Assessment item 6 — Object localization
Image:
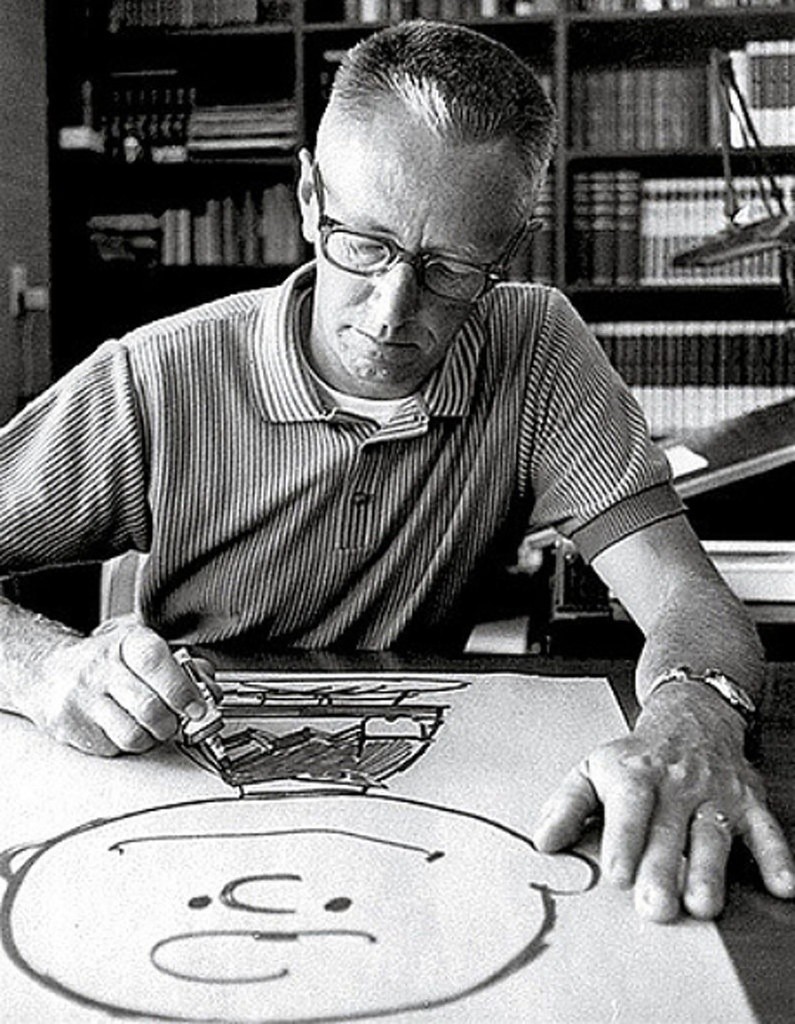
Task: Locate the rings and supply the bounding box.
[693,807,732,843]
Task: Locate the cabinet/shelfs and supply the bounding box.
[44,0,795,530]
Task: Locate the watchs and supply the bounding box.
[648,667,756,733]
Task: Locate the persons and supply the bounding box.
[0,27,795,923]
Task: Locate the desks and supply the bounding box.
[0,651,795,1024]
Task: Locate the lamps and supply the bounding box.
[674,46,795,268]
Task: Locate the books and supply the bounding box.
[53,1,795,440]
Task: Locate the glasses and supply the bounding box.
[310,165,530,305]
